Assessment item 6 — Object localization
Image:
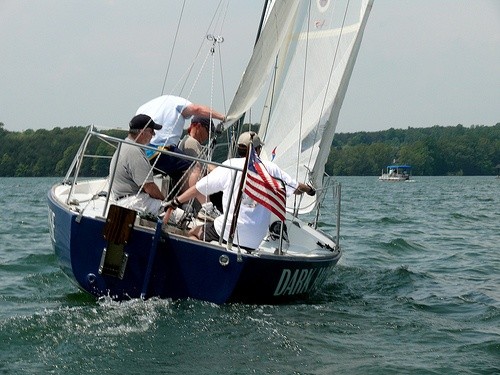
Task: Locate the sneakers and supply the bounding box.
[197,206,222,222]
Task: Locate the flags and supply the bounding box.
[243,142,287,222]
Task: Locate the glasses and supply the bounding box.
[149,130,155,136]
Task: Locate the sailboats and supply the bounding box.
[46,0,374,304]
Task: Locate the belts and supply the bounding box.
[117,190,143,201]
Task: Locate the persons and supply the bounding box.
[109,114,207,228]
[135,95,227,219]
[163,131,316,253]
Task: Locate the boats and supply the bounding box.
[378,165,412,181]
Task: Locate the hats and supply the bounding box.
[191,114,215,134]
[237,131,264,150]
[129,113,163,130]
